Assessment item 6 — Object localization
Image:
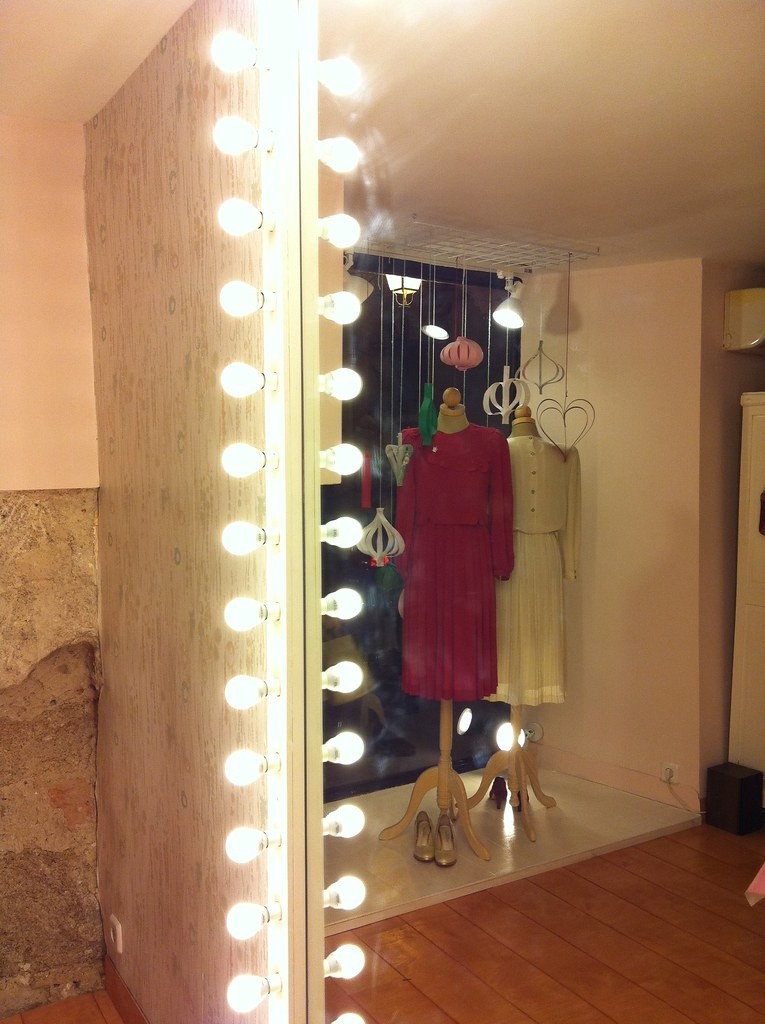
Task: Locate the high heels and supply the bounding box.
[517,791,529,812]
[414,811,435,862]
[435,815,457,865]
[489,777,507,808]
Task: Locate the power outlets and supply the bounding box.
[658,762,678,785]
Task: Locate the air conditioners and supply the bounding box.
[723,288,765,356]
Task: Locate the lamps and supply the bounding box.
[496,722,543,752]
[420,287,458,339]
[384,274,420,305]
[492,273,526,328]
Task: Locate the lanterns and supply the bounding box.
[439,335,484,372]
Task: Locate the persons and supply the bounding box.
[483,404,583,708]
[392,387,515,706]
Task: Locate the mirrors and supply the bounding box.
[304,0,765,1024]
